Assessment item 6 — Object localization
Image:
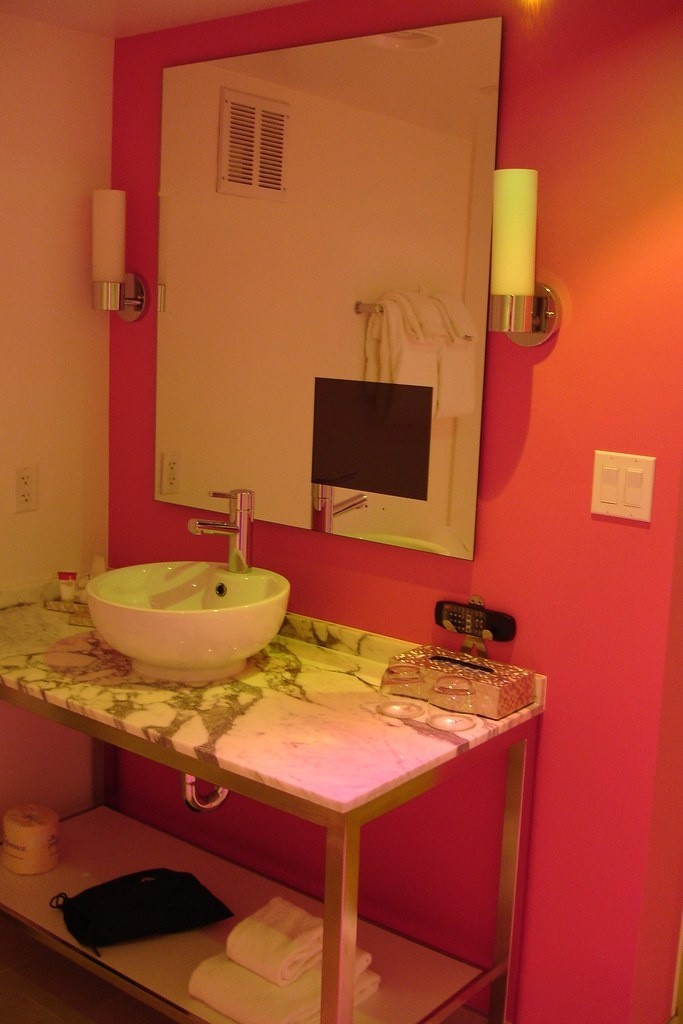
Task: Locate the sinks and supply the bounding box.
[88,561,290,682]
[345,535,450,560]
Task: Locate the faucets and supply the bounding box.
[189,488,254,573]
[310,484,368,533]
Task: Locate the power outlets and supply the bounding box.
[14,465,38,512]
[160,451,180,494]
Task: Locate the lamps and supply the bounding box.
[488,167,561,347]
[92,190,147,322]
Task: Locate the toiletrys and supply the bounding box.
[57,570,78,603]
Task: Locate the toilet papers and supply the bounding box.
[3,802,60,876]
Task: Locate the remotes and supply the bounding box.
[434,600,515,642]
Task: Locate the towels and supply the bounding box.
[365,290,481,416]
[188,896,382,1022]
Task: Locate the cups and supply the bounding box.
[378,665,427,718]
[427,675,477,731]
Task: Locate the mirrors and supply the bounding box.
[156,16,502,561]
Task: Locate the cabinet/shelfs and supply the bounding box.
[0,603,546,1024]
